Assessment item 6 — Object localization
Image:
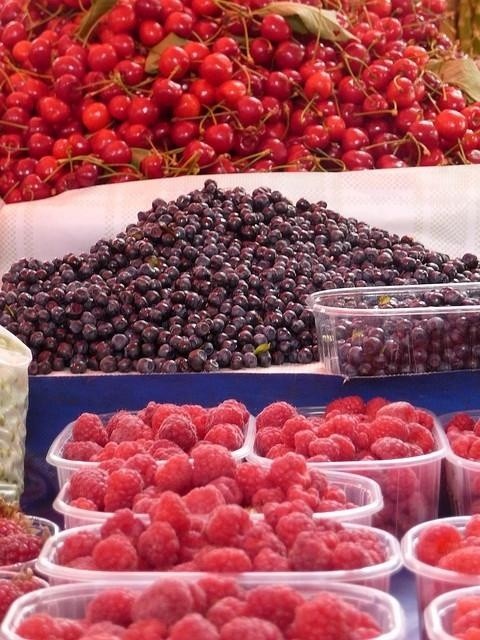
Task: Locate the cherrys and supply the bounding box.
[0,0,480,204]
